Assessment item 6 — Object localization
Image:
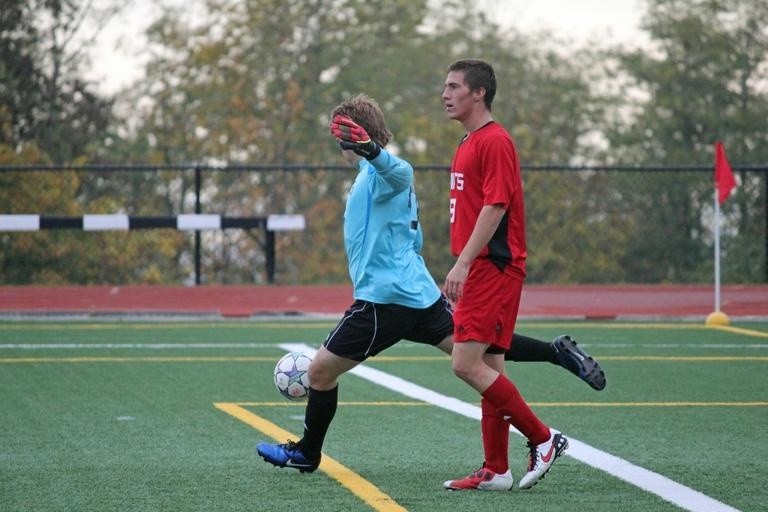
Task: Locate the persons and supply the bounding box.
[441,59,569,492]
[254,95,609,475]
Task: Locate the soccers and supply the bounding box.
[274,352,315,400]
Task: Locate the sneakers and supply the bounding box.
[443,466,515,491]
[255,439,325,473]
[546,332,607,392]
[518,423,570,490]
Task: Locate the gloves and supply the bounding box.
[329,112,381,162]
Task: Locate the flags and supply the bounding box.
[715,141,739,206]
